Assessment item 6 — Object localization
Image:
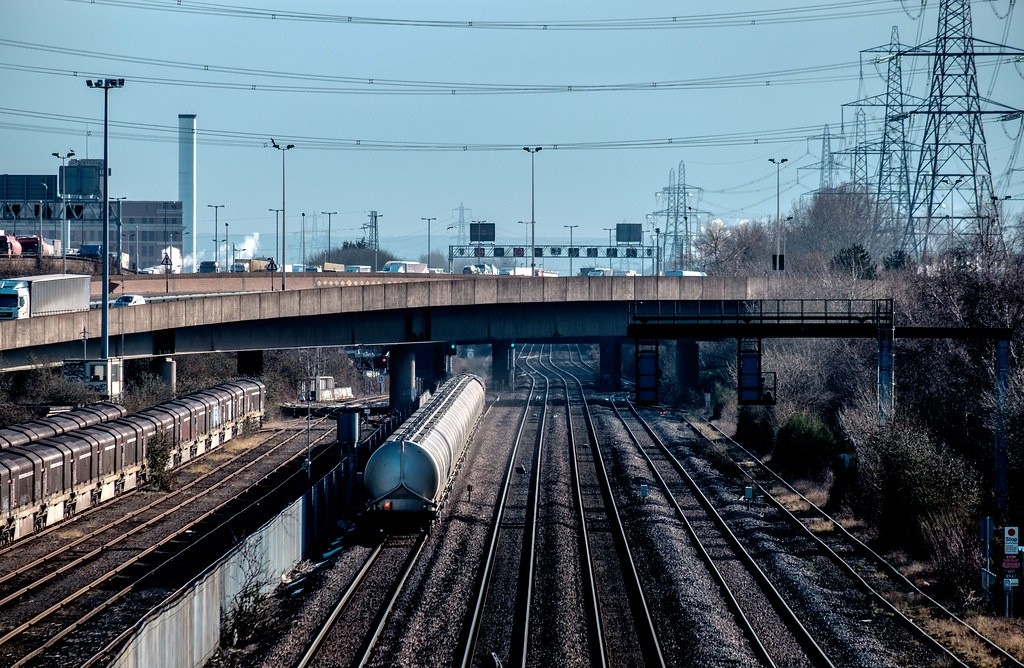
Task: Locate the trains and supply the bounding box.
[355,369,488,535]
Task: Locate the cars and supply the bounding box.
[112,294,146,309]
[230,263,246,272]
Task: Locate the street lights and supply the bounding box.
[160,201,190,274]
[684,216,687,271]
[521,145,544,277]
[655,227,660,274]
[235,248,246,263]
[367,214,383,271]
[603,228,617,268]
[207,204,226,273]
[52,152,76,275]
[768,157,793,300]
[471,220,487,265]
[86,78,125,360]
[688,206,692,270]
[321,211,338,263]
[421,217,436,268]
[564,225,579,277]
[268,144,295,272]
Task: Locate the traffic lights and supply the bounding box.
[379,356,387,365]
[447,342,457,356]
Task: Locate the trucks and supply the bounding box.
[0,235,132,321]
[292,260,643,277]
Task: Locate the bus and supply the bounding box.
[197,260,220,274]
